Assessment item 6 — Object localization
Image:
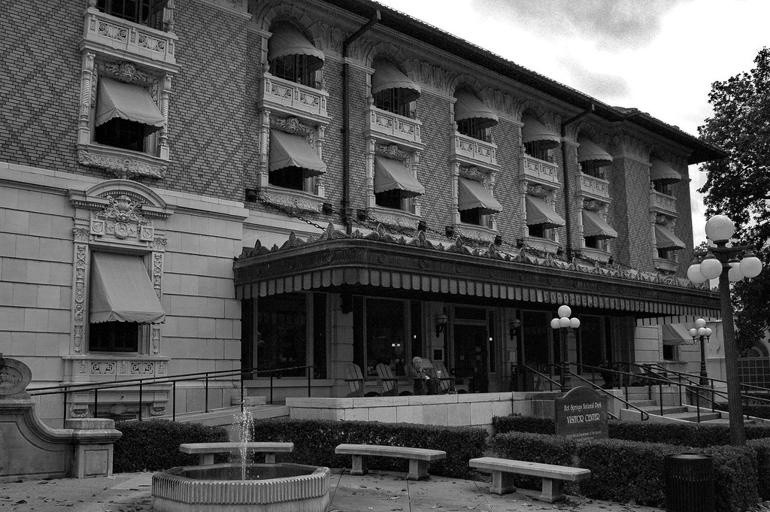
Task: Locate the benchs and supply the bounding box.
[179,442,294,465]
[469,457,591,503]
[335,443,446,479]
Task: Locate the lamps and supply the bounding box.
[436,315,449,337]
[510,319,521,340]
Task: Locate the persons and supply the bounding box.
[410,356,434,394]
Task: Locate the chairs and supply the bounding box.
[344,361,458,397]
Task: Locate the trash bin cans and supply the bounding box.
[662,451,714,512]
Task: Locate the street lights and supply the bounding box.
[686,214,763,445]
[689,317,713,385]
[551,305,581,393]
